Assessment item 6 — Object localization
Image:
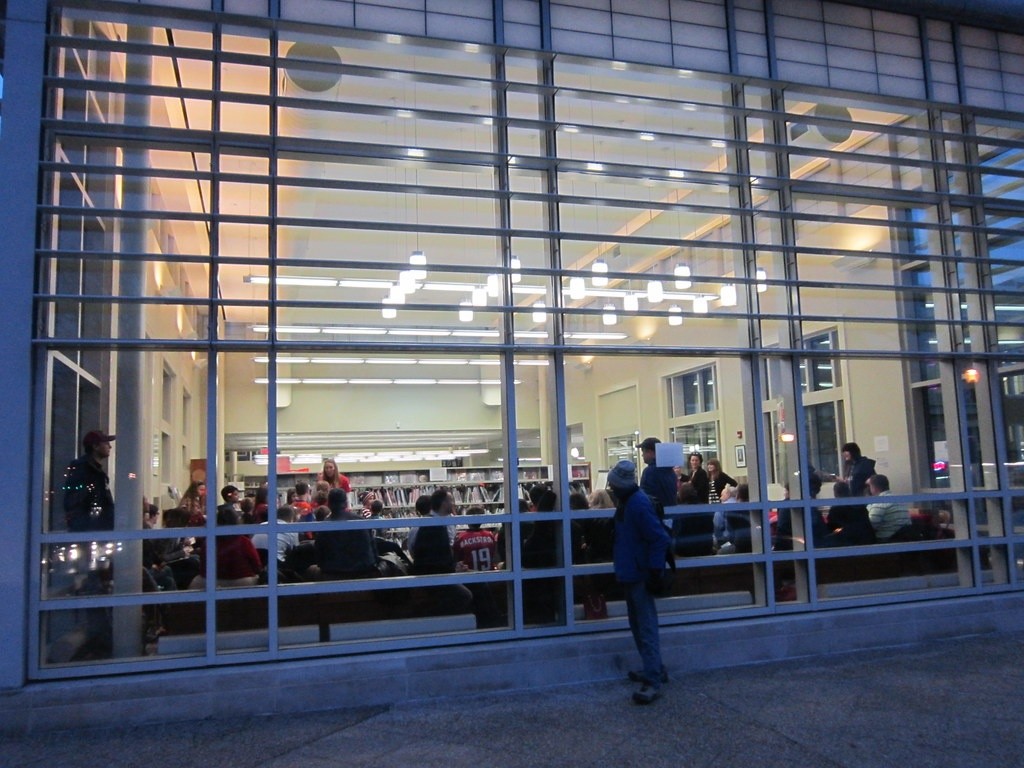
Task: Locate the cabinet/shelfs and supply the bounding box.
[245,461,592,534]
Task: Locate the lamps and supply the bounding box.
[242,54,769,385]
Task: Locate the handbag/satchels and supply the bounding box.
[582,595,609,619]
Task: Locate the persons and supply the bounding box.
[65,430,912,605]
[607,460,672,705]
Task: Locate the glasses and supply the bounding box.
[691,452,699,455]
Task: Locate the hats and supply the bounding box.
[83,429,116,444]
[220,485,238,501]
[635,437,661,451]
[607,461,636,489]
[357,491,374,506]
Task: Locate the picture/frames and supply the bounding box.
[735,444,747,468]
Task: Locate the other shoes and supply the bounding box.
[628,670,668,683]
[632,683,660,701]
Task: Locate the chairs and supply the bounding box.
[110,508,950,632]
